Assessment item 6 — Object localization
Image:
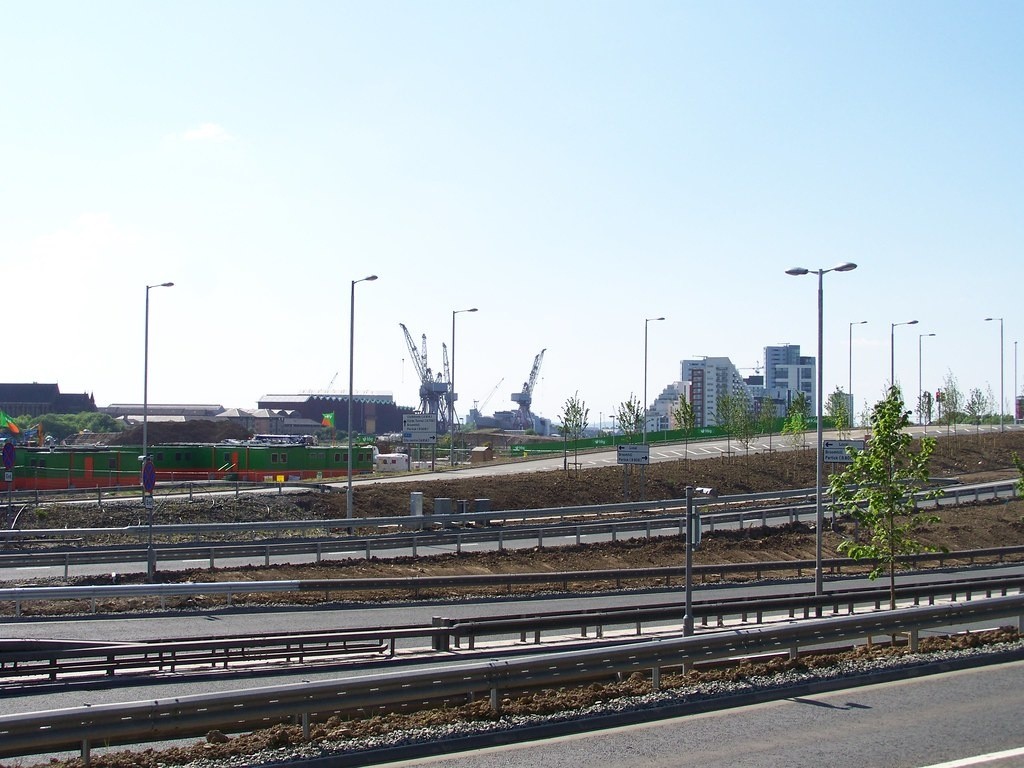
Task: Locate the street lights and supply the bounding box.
[786,261,857,613]
[891,320,919,392]
[918,333,936,425]
[850,321,867,428]
[141,281,174,500]
[985,318,1004,433]
[1015,341,1018,424]
[450,307,478,466]
[344,275,379,535]
[644,317,666,445]
[609,415,615,446]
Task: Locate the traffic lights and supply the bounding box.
[935,391,940,402]
[941,392,945,400]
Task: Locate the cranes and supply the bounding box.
[476,377,505,418]
[509,348,548,429]
[399,322,463,434]
[737,360,764,377]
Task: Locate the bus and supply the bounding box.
[250,433,326,444]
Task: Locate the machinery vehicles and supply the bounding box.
[16,422,44,452]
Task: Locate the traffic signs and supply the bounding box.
[823,440,864,463]
[617,444,650,465]
